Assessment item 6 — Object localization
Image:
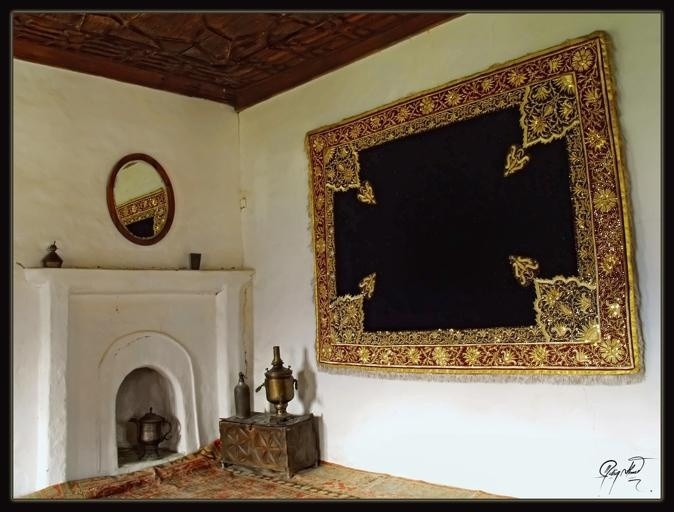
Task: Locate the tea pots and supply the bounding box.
[128,406,173,461]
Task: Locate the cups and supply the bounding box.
[190,252,201,270]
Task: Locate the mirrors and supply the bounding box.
[107,152,176,246]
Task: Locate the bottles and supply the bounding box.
[233,372,251,419]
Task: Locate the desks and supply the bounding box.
[218,409,319,478]
